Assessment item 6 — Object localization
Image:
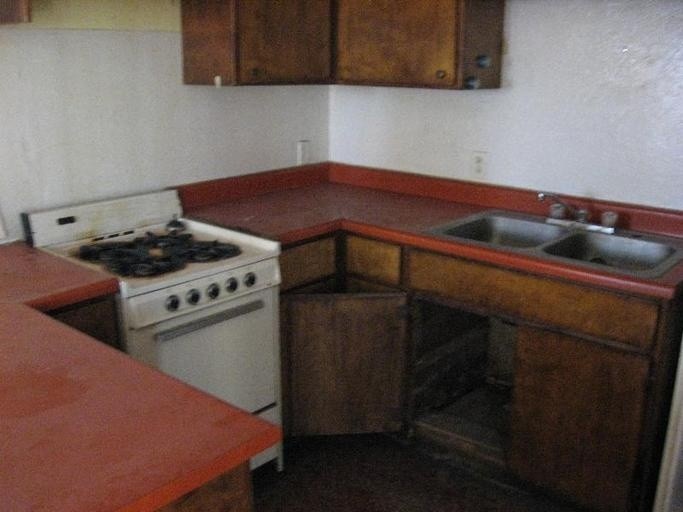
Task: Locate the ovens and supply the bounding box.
[127,259,283,414]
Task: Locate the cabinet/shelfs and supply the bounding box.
[289,277,651,512]
[179,0,505,90]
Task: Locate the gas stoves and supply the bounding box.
[79,216,242,274]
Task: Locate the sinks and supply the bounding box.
[540,224,683,280]
[421,208,577,254]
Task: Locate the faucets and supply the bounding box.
[537,193,594,223]
